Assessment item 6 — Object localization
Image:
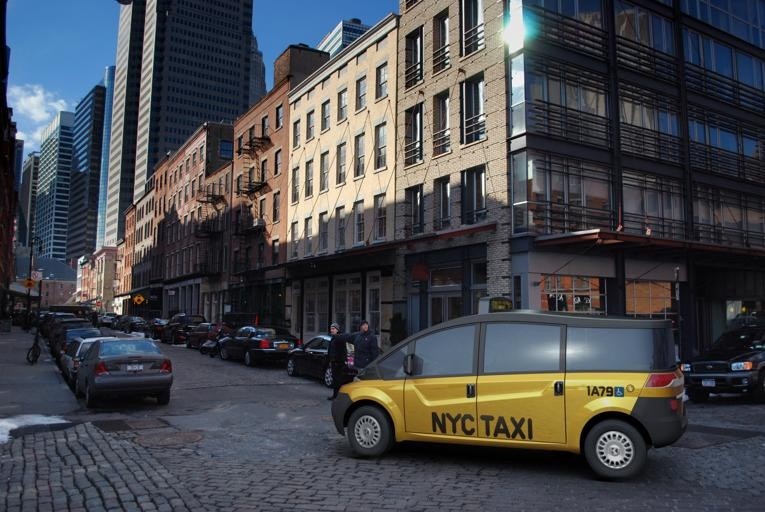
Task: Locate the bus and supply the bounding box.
[49,304,94,321]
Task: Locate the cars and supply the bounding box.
[111,315,169,339]
[55,327,173,408]
[185,321,298,366]
[286,336,385,387]
[680,325,765,405]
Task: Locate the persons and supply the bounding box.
[342,320,380,369]
[327,321,347,400]
[92,310,98,327]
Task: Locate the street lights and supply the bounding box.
[24,235,43,330]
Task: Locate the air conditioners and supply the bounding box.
[252,217,265,226]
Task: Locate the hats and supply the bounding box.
[331,322,340,331]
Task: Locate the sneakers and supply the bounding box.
[328,396,335,400]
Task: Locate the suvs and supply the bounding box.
[160,312,207,345]
[97,312,117,328]
[38,311,91,356]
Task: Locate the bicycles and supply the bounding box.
[27,331,41,364]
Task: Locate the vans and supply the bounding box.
[331,310,687,478]
